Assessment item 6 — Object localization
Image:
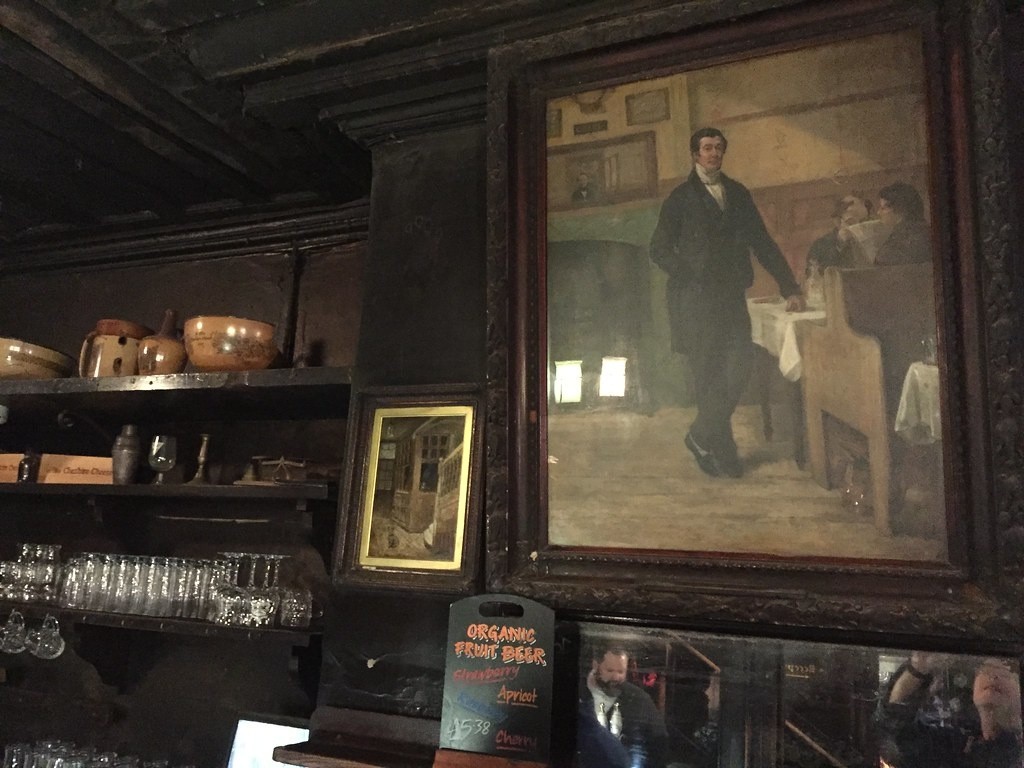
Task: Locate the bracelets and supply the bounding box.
[906,658,933,680]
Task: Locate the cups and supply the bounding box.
[77,318,157,381]
[63,549,227,622]
[11,543,62,603]
[3,736,195,767]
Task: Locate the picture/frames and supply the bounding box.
[329,380,487,595]
[473,0,1014,648]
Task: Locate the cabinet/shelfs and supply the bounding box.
[0,367,352,650]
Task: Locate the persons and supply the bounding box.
[874,650,1024,768]
[579,640,719,768]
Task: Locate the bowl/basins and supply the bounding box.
[0,337,76,386]
[183,316,281,372]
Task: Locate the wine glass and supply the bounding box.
[213,548,313,629]
[148,435,177,488]
